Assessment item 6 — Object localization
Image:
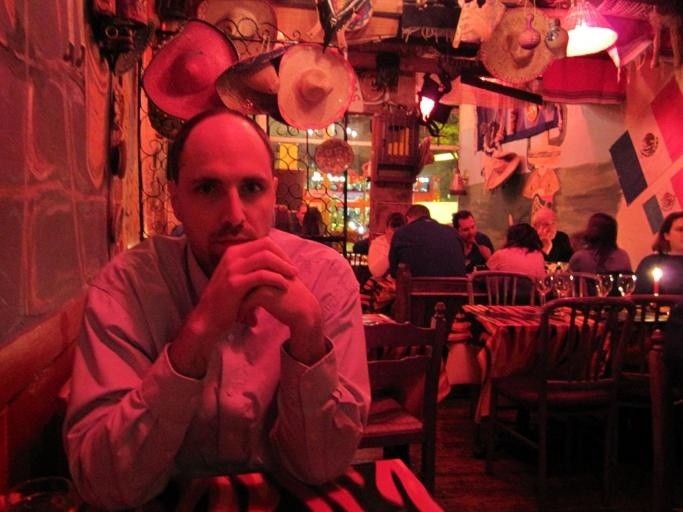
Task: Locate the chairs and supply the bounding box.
[51,261,683,512]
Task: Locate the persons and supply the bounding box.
[368,204,683,303]
[59,106,373,512]
[292,199,324,240]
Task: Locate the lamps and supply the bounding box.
[561,0,618,58]
[420,85,443,125]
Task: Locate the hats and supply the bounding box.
[477,6,554,83]
[143,16,241,119]
[215,41,357,130]
[196,0,278,64]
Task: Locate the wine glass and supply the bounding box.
[536,274,638,320]
